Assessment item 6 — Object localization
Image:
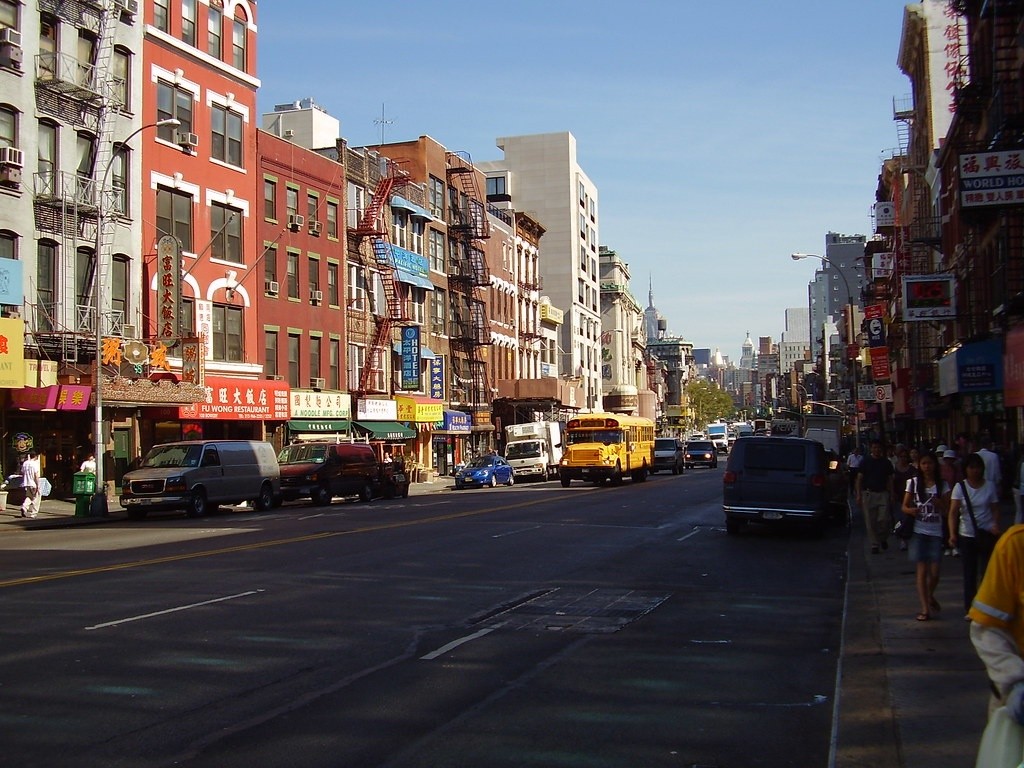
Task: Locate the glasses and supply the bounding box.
[899,455,907,458]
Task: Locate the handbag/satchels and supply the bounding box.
[974,529,1002,565]
[891,478,915,539]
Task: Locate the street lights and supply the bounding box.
[94,118,181,488]
[791,251,861,449]
[787,383,809,413]
[588,328,624,412]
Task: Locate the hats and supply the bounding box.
[943,450,955,458]
[935,445,947,452]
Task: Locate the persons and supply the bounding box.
[80,453,96,475]
[847,431,1024,768]
[384,452,392,463]
[20,451,41,519]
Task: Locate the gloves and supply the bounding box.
[1006,683,1024,724]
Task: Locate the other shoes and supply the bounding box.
[944,549,950,556]
[871,546,879,554]
[952,549,960,557]
[30,514,37,518]
[897,540,906,551]
[20,507,27,518]
[881,539,888,550]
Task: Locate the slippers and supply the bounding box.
[929,597,940,614]
[916,613,928,621]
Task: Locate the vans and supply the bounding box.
[119,440,281,515]
[722,436,841,538]
[276,441,382,506]
[649,437,684,475]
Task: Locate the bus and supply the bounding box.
[558,414,656,487]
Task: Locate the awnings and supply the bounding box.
[288,419,348,431]
[393,341,436,360]
[391,195,434,222]
[393,270,434,291]
[352,421,416,440]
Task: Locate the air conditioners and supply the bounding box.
[120,0,139,16]
[265,281,279,293]
[178,132,198,147]
[430,208,440,218]
[0,27,23,45]
[310,378,325,389]
[310,290,322,300]
[448,266,459,274]
[0,147,24,167]
[435,324,442,333]
[309,220,325,233]
[120,324,136,338]
[291,214,305,227]
[267,375,285,380]
[285,129,294,136]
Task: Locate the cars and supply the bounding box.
[825,446,849,509]
[455,455,515,490]
[683,429,718,469]
[728,424,771,446]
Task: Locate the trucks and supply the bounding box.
[708,423,728,454]
[804,412,842,457]
[504,421,563,483]
